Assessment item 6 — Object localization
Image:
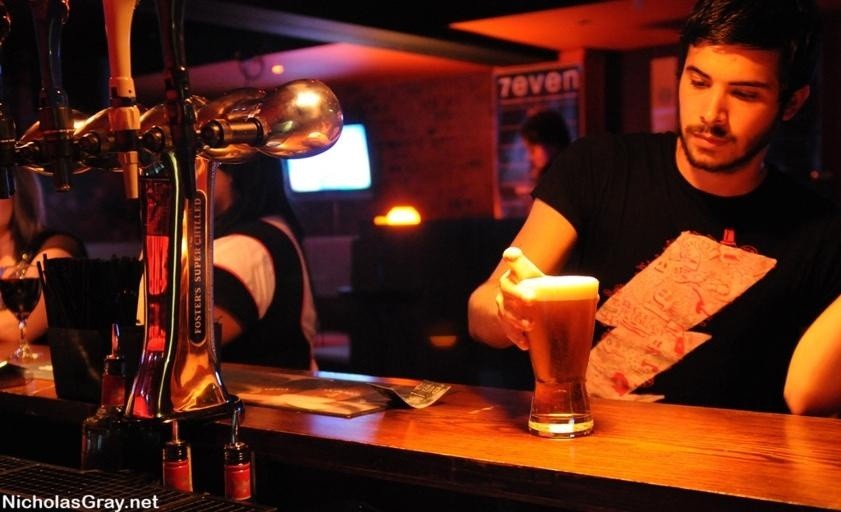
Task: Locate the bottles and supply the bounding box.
[221,403,261,502]
[163,421,195,495]
[98,321,128,409]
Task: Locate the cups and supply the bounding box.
[515,274,604,438]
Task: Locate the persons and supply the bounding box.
[137,150,320,370]
[0,167,90,344]
[520,102,572,181]
[465,0,841,417]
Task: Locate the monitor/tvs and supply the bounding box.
[281,119,375,198]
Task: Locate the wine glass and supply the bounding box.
[0,264,46,365]
